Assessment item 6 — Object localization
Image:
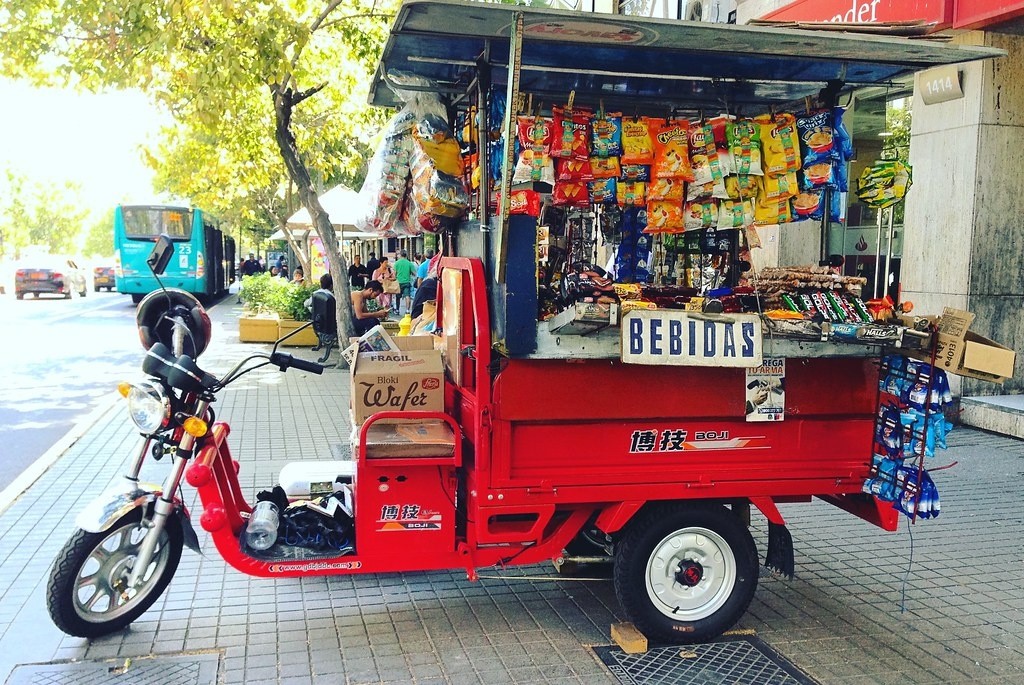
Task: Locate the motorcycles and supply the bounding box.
[45,0,1008,646]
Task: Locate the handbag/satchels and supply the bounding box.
[414,280,417,288]
[382,278,400,294]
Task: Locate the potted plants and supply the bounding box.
[238,271,290,342]
[276,283,385,347]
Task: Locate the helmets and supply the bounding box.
[136,287,212,359]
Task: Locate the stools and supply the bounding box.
[349,411,465,458]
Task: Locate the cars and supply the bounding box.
[14,254,87,300]
[93,262,117,292]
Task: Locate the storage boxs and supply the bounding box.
[349,335,444,426]
[896,307,1016,384]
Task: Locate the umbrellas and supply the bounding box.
[268,184,420,261]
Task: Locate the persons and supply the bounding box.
[277,265,289,280]
[348,255,369,289]
[372,257,395,321]
[367,252,380,282]
[411,260,442,336]
[304,274,334,313]
[746,388,768,414]
[276,256,285,275]
[289,269,303,285]
[410,253,423,314]
[393,249,416,315]
[417,248,434,289]
[351,280,389,337]
[238,258,244,281]
[269,266,276,277]
[759,377,786,392]
[241,253,261,276]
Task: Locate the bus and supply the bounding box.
[112,204,235,302]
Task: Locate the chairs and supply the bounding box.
[310,289,337,363]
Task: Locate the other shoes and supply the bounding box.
[393,309,399,315]
[406,311,410,314]
